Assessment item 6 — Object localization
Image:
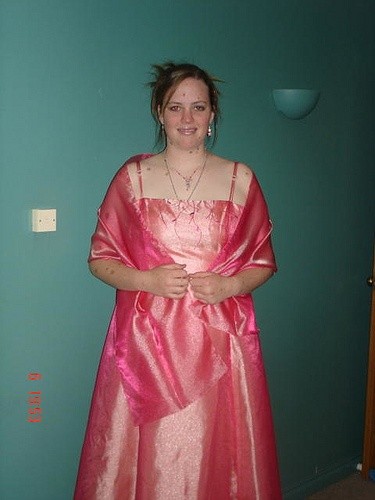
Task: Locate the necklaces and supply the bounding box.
[158,151,209,212]
[162,152,206,190]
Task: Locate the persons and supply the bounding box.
[74,61,283,500]
